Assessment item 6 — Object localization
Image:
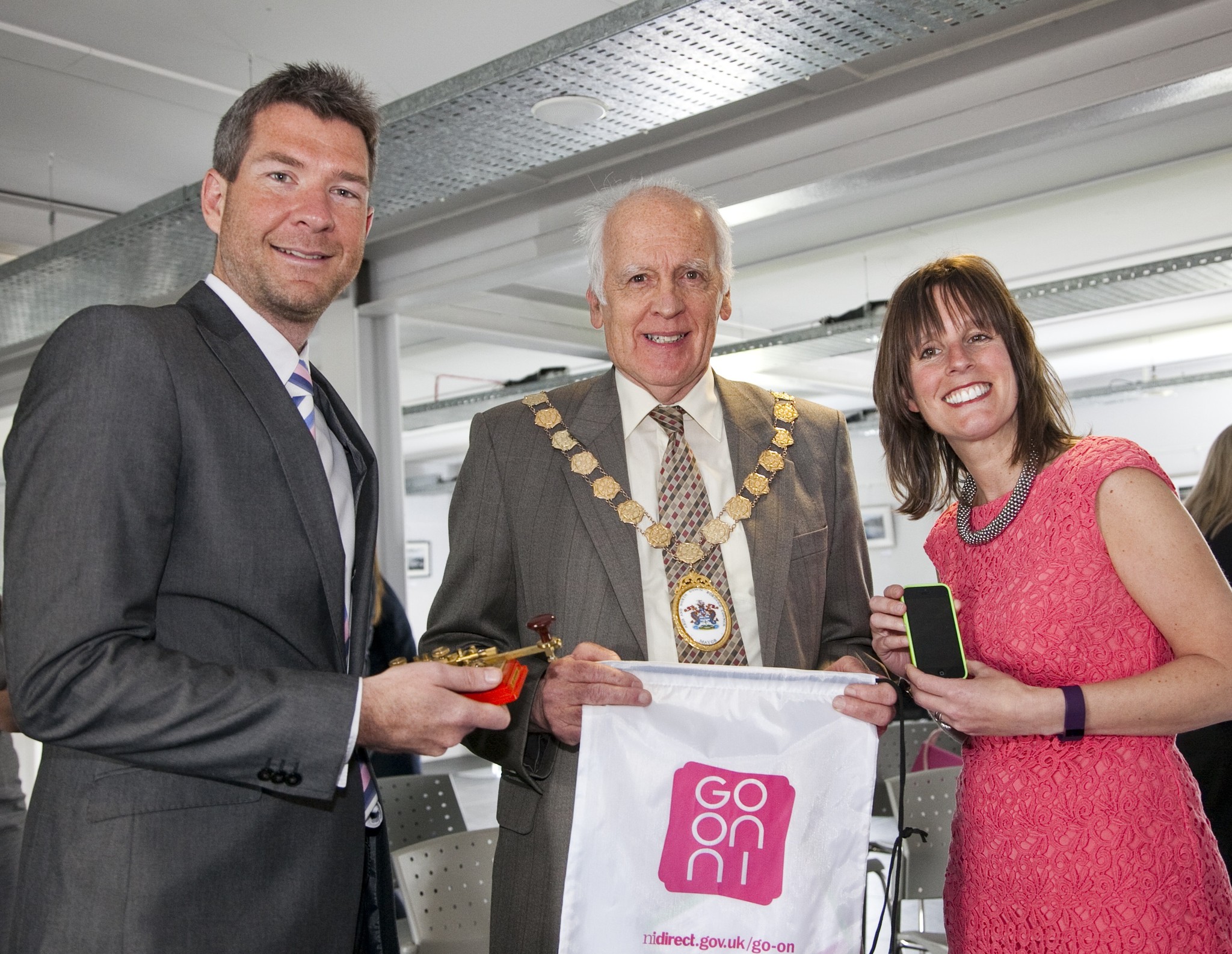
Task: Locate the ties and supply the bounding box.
[652,406,749,665]
[287,359,382,827]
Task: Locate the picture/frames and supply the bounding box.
[405,540,431,580]
[861,504,897,551]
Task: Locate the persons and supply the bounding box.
[365,569,422,777]
[0,59,512,954]
[872,257,1232,954]
[416,183,903,954]
[1173,425,1232,868]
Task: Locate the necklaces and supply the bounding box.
[957,436,1041,545]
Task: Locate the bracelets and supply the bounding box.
[1057,684,1086,741]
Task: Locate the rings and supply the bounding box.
[933,711,942,722]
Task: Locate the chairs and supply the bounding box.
[378,773,469,920]
[867,858,948,954]
[875,719,962,817]
[389,826,500,954]
[886,766,963,932]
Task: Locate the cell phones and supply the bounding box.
[900,582,968,680]
